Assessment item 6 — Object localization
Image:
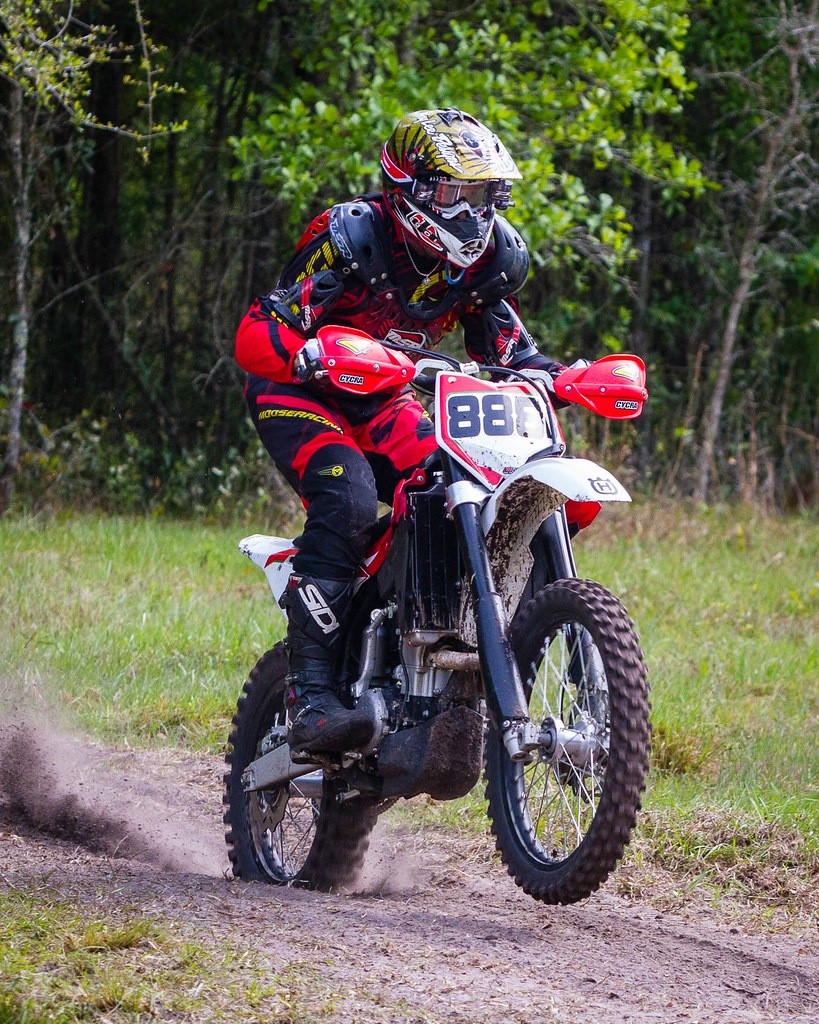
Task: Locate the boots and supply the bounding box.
[278,572,376,757]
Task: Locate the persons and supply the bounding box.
[235,104,575,765]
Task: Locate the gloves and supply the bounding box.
[293,338,322,382]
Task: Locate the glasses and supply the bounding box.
[431,179,490,219]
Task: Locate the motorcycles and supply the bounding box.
[220,326,651,907]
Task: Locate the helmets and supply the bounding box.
[380,106,524,269]
